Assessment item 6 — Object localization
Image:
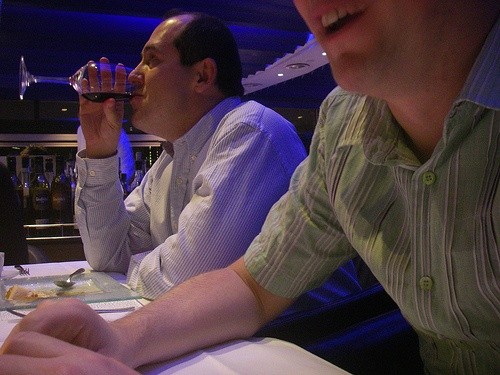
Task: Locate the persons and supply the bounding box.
[75,11,362,314]
[0,0,500,375]
[0,162,29,265]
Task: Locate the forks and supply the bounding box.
[14,264,30,276]
[5,306,134,318]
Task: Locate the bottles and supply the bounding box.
[128,161,145,193]
[4,155,76,237]
[134,145,162,173]
[119,173,129,201]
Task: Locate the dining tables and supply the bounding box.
[0,261,352,375]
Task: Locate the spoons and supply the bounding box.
[53,268,85,288]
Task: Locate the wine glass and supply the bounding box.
[17,55,139,103]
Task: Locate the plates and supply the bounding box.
[0,272,140,311]
